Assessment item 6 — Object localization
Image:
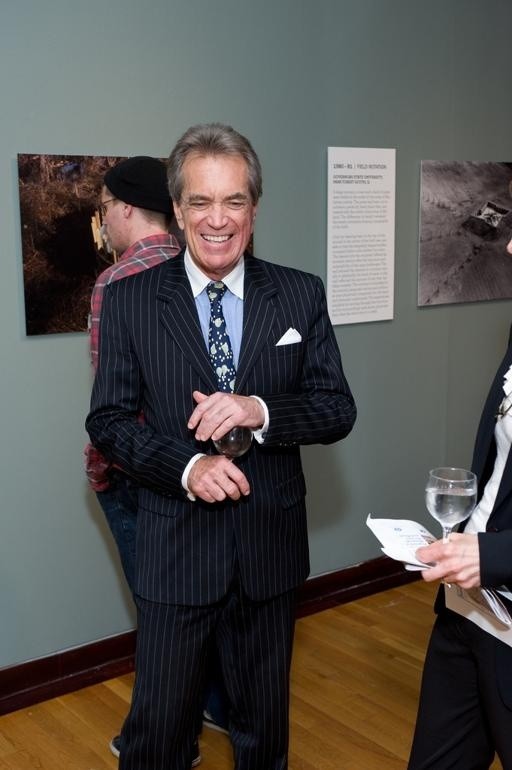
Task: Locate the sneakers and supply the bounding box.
[111,732,203,767]
[201,711,230,738]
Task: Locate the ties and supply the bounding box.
[205,281,236,396]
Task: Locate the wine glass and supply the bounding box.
[423,466,480,586]
[212,420,253,465]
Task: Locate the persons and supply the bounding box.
[404,235,512,769]
[86,153,237,766]
[89,120,357,769]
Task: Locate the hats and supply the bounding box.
[104,155,174,214]
